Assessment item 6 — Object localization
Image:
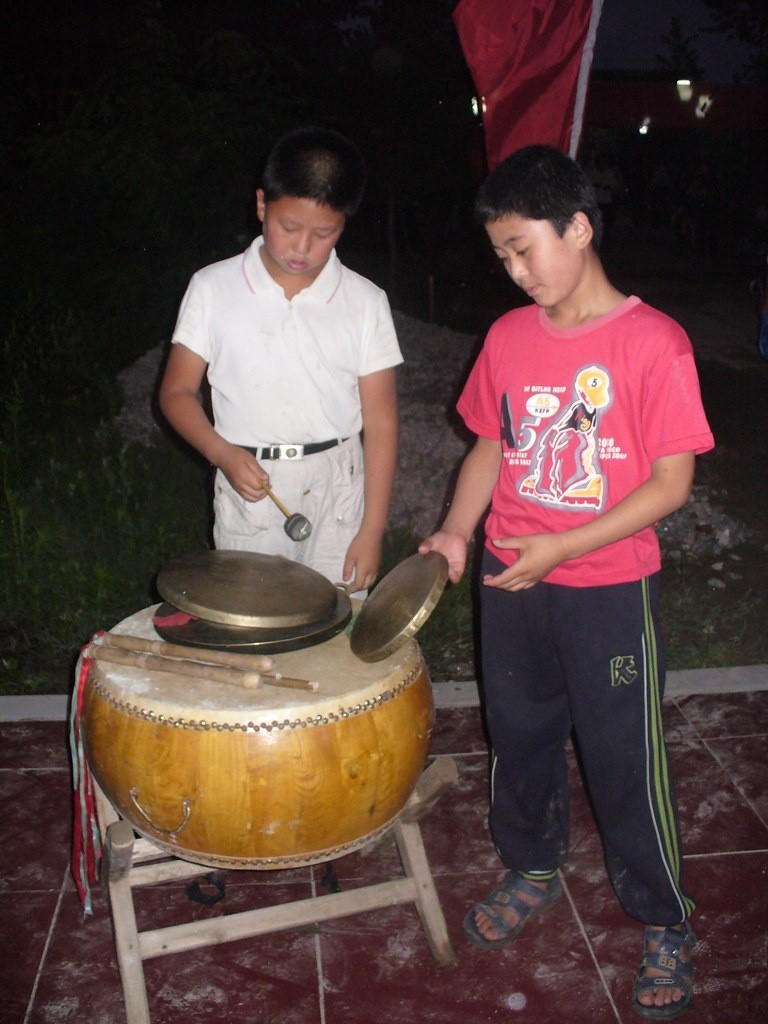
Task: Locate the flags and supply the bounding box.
[452,0,594,176]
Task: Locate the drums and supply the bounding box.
[72,595,437,873]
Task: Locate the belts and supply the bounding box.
[235,437,350,461]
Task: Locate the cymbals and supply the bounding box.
[151,549,353,655]
[349,550,449,664]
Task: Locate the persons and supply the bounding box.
[159,126,407,601]
[419,144,716,1017]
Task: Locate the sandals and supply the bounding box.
[463,871,563,949]
[632,919,696,1019]
